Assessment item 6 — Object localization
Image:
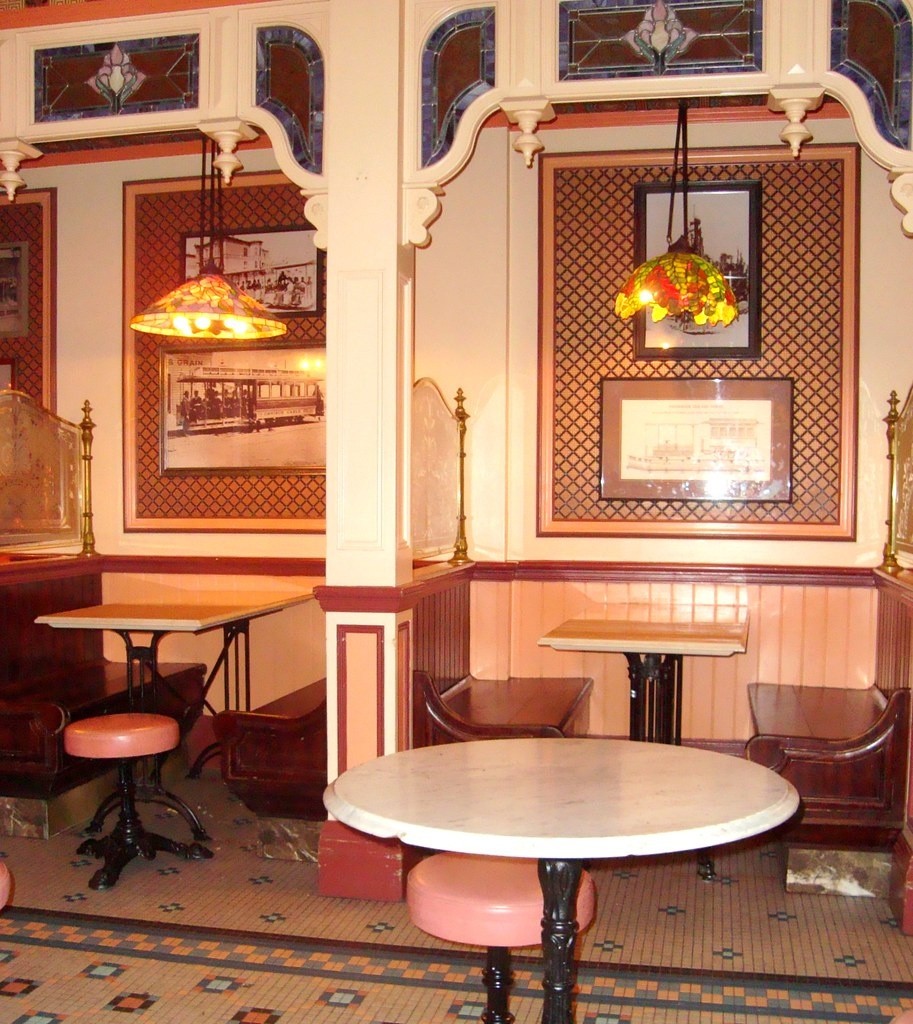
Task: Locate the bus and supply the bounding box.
[176,364,321,432]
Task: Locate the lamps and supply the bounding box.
[615,99,739,334]
[131,136,288,340]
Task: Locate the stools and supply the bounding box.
[407,852,595,1024]
[63,713,215,891]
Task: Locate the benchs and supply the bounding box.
[414,669,593,748]
[0,658,208,840]
[213,676,327,822]
[744,684,911,851]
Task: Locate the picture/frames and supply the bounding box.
[120,169,326,536]
[598,377,794,502]
[633,178,764,361]
[0,187,58,419]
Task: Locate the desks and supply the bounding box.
[33,590,316,841]
[323,739,798,1024]
[536,604,750,882]
